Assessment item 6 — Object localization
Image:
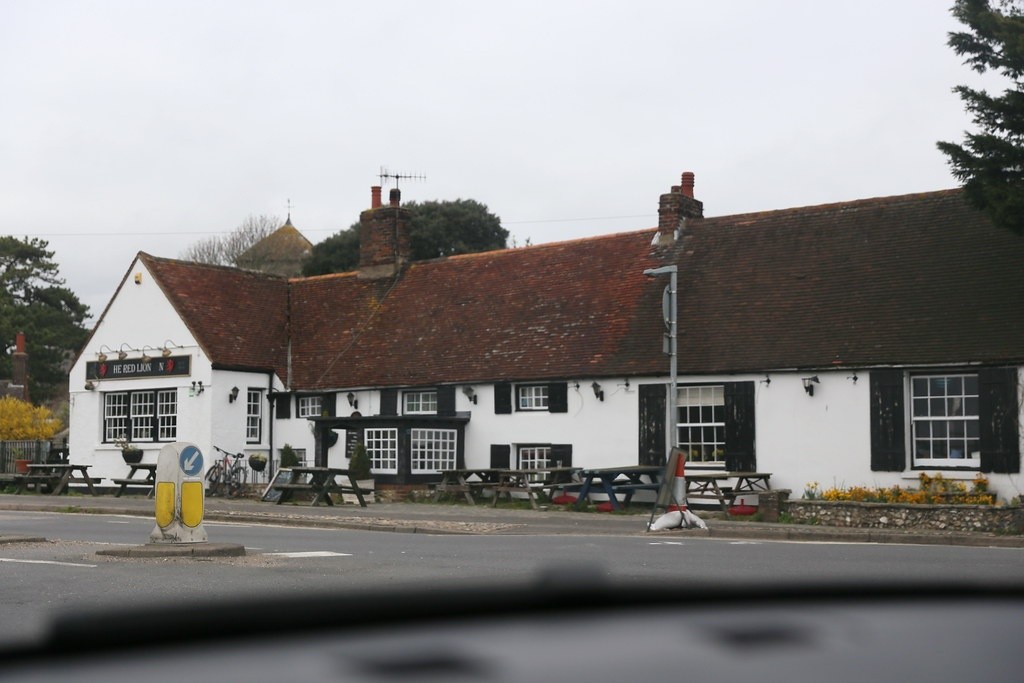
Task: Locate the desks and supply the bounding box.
[491,466,577,512]
[685,472,773,515]
[261,466,366,507]
[115,462,158,498]
[435,468,519,505]
[16,464,98,495]
[572,463,665,513]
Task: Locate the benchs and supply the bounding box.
[14,476,159,484]
[428,478,770,497]
[269,483,377,494]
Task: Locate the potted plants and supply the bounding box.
[342,442,377,506]
[309,411,338,449]
[14,449,33,474]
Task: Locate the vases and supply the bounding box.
[249,460,265,471]
[122,450,145,463]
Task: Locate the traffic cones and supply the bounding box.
[668,453,689,514]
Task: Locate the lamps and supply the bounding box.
[592,381,604,402]
[347,391,357,410]
[162,339,184,356]
[98,344,117,362]
[463,385,477,405]
[802,377,813,397]
[85,363,105,392]
[142,345,154,363]
[119,342,140,360]
[229,386,239,405]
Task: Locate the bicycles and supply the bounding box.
[204,446,249,498]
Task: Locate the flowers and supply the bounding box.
[250,454,266,462]
[116,436,142,452]
